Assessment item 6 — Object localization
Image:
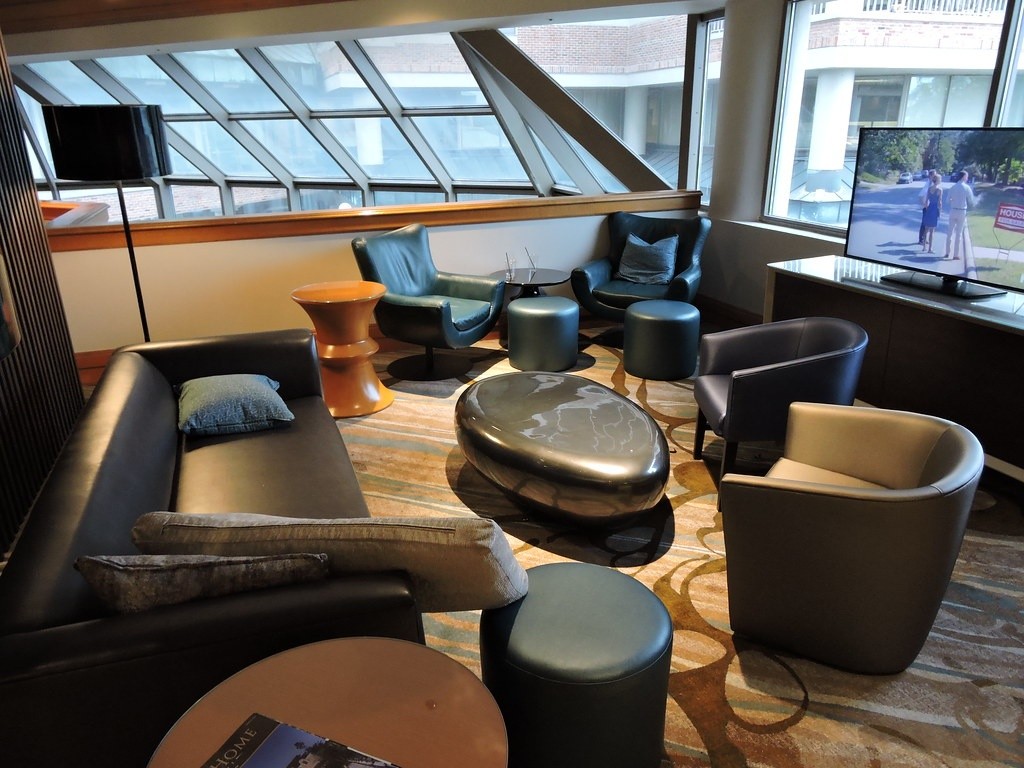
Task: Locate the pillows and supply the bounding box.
[611,232,682,286]
[130,511,530,613]
[171,374,295,437]
[73,552,330,617]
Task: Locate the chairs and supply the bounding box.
[694,317,868,511]
[351,223,505,381]
[571,209,712,323]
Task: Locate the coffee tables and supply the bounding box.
[454,369,670,518]
[146,635,510,768]
[290,280,394,420]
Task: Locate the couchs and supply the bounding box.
[720,400,984,676]
[0,332,428,768]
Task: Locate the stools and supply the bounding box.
[478,562,673,768]
[623,300,700,381]
[508,296,580,372]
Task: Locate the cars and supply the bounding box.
[950,173,960,182]
[898,172,913,184]
[912,171,923,181]
[921,169,929,178]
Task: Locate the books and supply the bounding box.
[200,712,402,768]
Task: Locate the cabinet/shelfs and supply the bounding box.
[761,254,1024,505]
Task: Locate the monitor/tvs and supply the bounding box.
[843,128,1024,299]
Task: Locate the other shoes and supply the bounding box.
[953,256,960,259]
[944,255,949,258]
[918,240,930,245]
[923,247,928,251]
[928,249,935,253]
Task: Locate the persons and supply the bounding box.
[943,171,981,260]
[922,174,942,254]
[919,169,936,245]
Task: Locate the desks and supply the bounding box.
[490,268,571,302]
